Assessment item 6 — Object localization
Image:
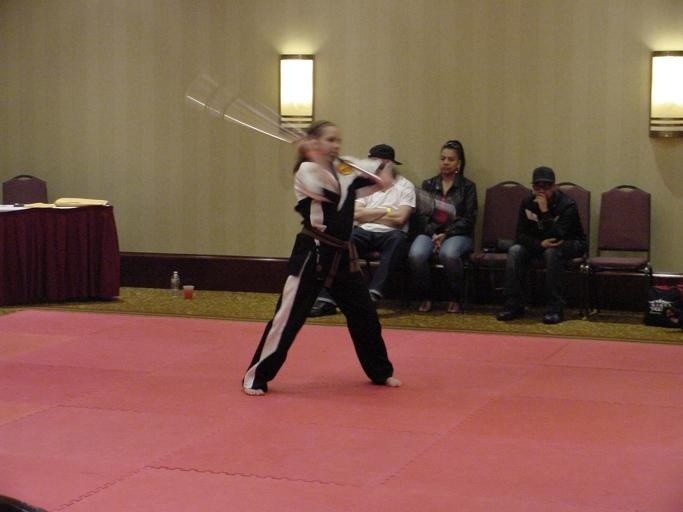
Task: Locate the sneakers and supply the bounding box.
[496,308,526,322]
[418,301,431,313]
[309,301,337,317]
[543,309,565,324]
[447,301,460,313]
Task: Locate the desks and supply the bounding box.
[0,199,125,309]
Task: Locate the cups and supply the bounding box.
[182,286,193,299]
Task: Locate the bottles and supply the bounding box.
[169,271,180,299]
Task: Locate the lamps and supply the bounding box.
[275,51,317,137]
[647,44,683,144]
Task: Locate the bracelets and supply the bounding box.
[386,208,392,217]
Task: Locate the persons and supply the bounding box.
[497,167,587,324]
[407,140,478,313]
[242,120,401,396]
[309,145,417,318]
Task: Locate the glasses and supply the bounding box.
[533,183,553,191]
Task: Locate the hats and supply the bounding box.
[368,144,403,165]
[532,167,555,184]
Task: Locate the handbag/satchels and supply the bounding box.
[482,238,514,253]
[645,298,680,328]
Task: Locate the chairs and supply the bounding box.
[2,173,48,206]
[528,178,591,324]
[585,181,657,323]
[464,179,535,313]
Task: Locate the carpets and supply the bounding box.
[0,301,682,512]
